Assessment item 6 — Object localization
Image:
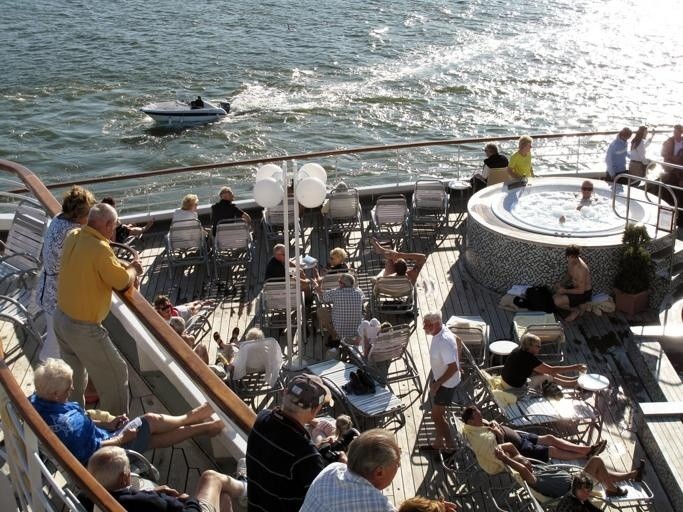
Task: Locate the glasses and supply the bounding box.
[161,305,170,311]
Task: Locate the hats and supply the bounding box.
[286,373,327,408]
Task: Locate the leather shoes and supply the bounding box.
[606,486,628,497]
[635,459,646,482]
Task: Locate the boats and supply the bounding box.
[137,97,230,126]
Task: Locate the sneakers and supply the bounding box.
[586,440,607,460]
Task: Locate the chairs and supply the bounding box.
[424,313,682,512]
[370,194,417,250]
[323,187,365,248]
[411,177,450,233]
[0,196,48,293]
[162,219,213,280]
[255,194,307,255]
[0,232,426,491]
[473,162,506,192]
[212,216,258,273]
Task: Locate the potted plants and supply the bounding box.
[612,221,656,317]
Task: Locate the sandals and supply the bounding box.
[230,327,239,343]
[214,332,223,348]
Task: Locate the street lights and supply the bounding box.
[250,151,328,373]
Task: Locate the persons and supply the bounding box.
[469,143,507,191]
[500,333,591,397]
[660,125,683,189]
[507,134,540,180]
[191,96,203,108]
[626,125,654,188]
[604,128,631,186]
[495,442,645,497]
[556,477,601,512]
[552,245,592,323]
[462,406,607,476]
[558,180,596,225]
[26,179,462,511]
